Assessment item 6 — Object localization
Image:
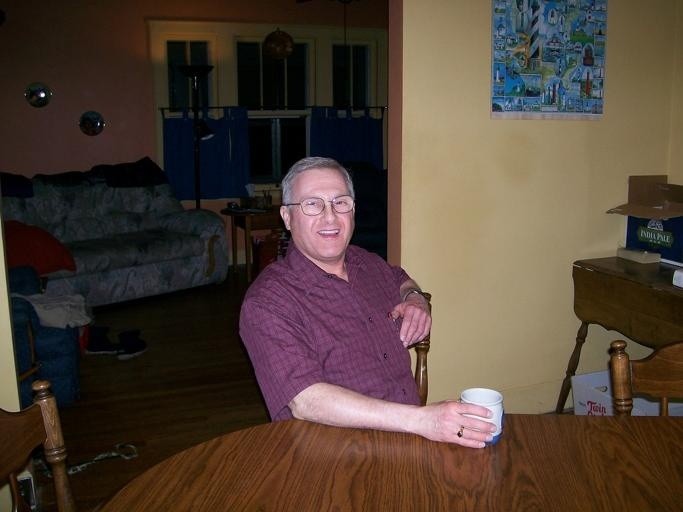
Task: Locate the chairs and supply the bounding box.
[2,268,82,411]
[0,380,77,509]
[610,340,683,413]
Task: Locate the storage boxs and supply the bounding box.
[604,175,683,268]
[570,368,683,416]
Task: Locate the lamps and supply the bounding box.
[264,26,295,56]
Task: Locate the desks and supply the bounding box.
[555,256,683,418]
[221,205,285,282]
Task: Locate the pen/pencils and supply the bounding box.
[388,313,392,319]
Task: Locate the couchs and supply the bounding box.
[0,156,230,307]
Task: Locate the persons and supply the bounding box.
[237,156,498,451]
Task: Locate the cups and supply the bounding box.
[460,388,504,446]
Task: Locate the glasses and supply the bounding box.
[282,195,356,216]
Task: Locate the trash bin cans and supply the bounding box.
[252,238,278,271]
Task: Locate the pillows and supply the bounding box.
[3,219,76,275]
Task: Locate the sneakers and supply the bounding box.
[86,327,148,360]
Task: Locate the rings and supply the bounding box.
[458,426,464,438]
